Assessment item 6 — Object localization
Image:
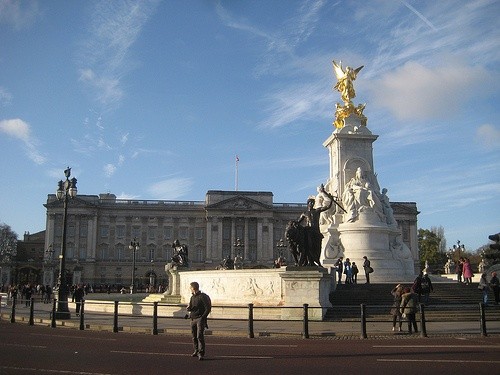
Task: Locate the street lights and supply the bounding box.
[55,167,78,318]
[452,241,465,259]
[128,237,140,295]
[232,239,244,256]
[276,238,288,257]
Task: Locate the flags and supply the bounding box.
[236,156,239,161]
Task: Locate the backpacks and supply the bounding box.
[190,292,211,317]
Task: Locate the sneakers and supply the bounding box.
[191,351,204,361]
[392,326,418,334]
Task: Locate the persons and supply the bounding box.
[455,256,474,286]
[219,253,244,269]
[171,240,187,267]
[186,281,211,360]
[315,168,397,225]
[333,66,357,105]
[71,285,85,316]
[0,281,169,307]
[478,272,500,305]
[294,193,334,266]
[273,255,286,268]
[333,255,371,284]
[389,272,433,334]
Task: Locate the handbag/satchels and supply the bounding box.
[368,267,373,273]
[478,285,485,290]
